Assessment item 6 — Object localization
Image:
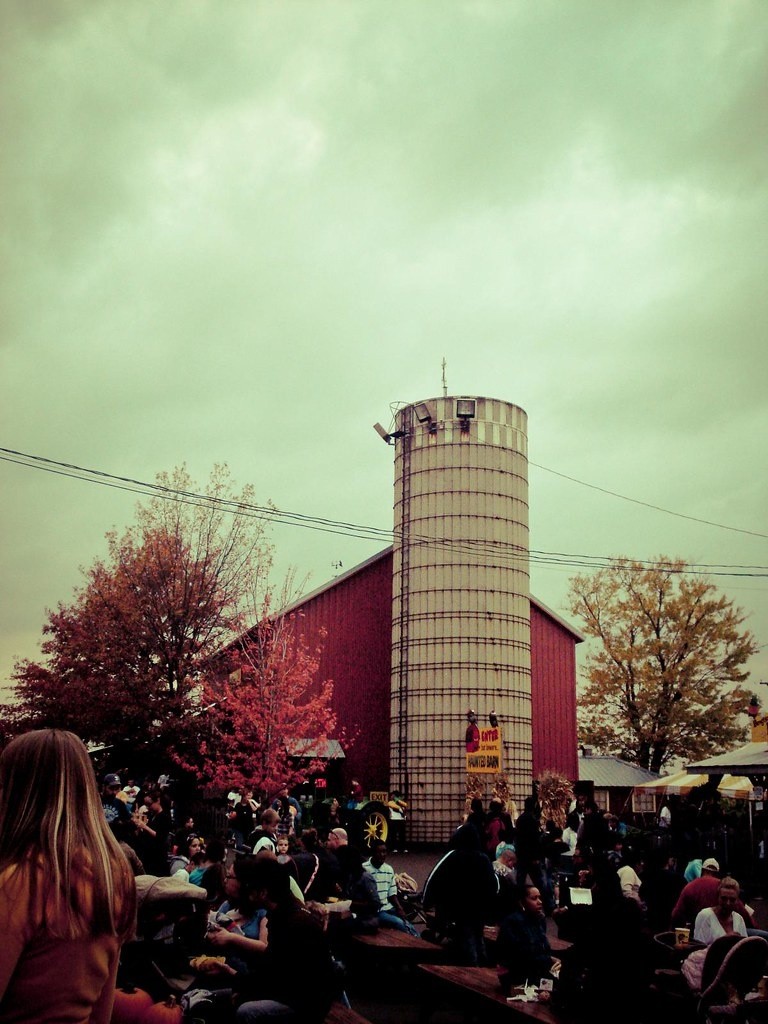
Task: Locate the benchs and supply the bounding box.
[418,962,559,1024]
[481,925,572,960]
[325,1003,371,1024]
[351,928,445,958]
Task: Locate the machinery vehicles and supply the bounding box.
[296,794,391,856]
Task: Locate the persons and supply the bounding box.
[0,729,138,1024]
[101,774,768,1024]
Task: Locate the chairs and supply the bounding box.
[653,927,768,1024]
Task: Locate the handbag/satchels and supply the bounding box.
[395,872,418,893]
[497,821,507,842]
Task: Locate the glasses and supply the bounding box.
[226,872,236,881]
[721,895,736,902]
[107,786,120,791]
[329,829,338,838]
[573,854,582,859]
[145,801,155,807]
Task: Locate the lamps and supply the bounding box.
[414,400,437,435]
[457,400,476,431]
[390,431,410,439]
[374,422,396,445]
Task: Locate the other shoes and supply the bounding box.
[405,920,420,938]
[392,849,398,852]
[403,849,408,853]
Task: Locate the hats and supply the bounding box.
[103,773,121,786]
[702,857,720,872]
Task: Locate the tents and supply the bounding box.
[633,770,755,831]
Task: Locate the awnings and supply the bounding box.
[283,739,345,758]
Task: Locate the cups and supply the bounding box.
[675,928,690,946]
